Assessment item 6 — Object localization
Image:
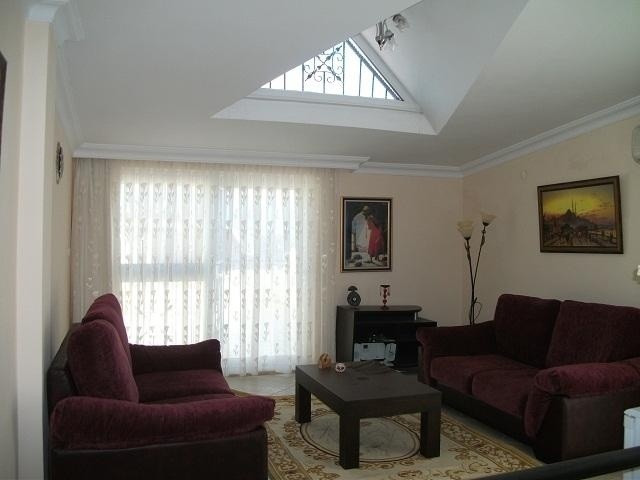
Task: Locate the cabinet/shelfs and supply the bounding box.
[336,305,436,373]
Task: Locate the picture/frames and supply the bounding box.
[537,176,624,254]
[340,196,393,273]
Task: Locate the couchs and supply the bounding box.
[47,293,275,480]
[416,293,640,463]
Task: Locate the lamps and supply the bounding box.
[376,15,409,52]
[457,213,495,324]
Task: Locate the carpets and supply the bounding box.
[231,388,547,480]
